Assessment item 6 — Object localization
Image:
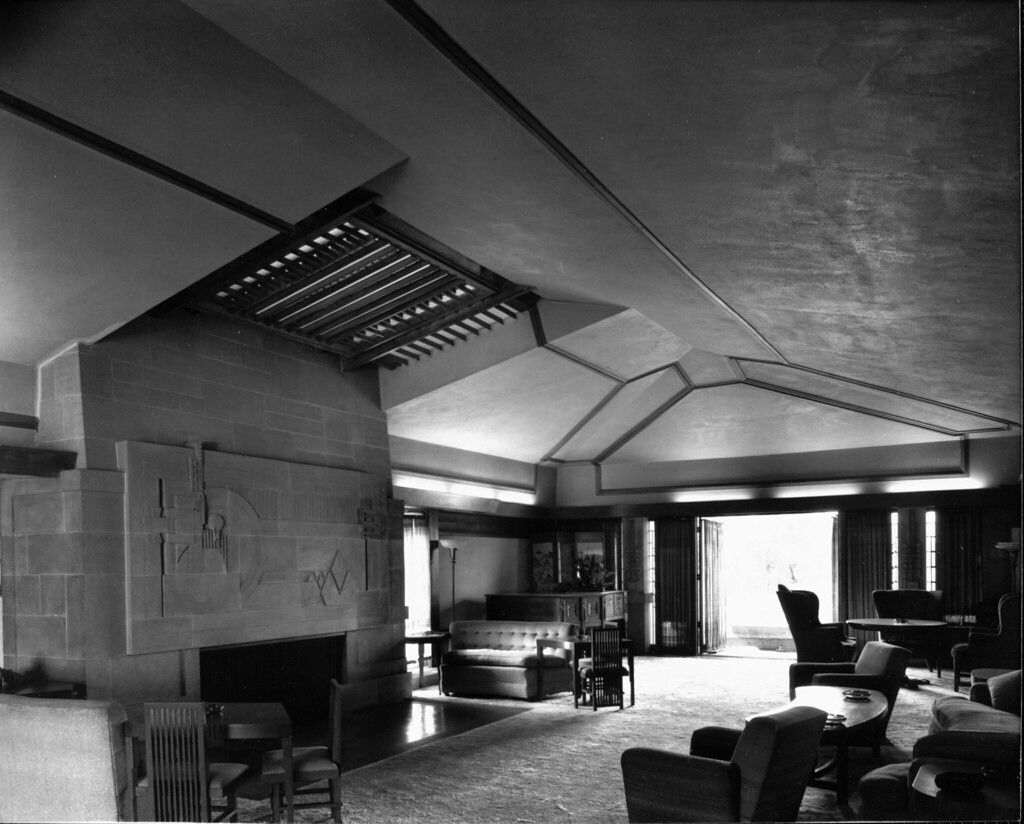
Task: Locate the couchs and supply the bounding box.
[620,705,826,824]
[437,620,576,699]
[858,668,1024,821]
[789,642,910,747]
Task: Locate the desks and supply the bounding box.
[402,631,449,694]
[122,701,294,824]
[845,615,948,687]
[968,665,1014,688]
[534,633,635,710]
[739,681,889,803]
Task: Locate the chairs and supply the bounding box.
[870,588,945,671]
[140,702,249,822]
[566,625,623,712]
[951,593,1022,689]
[773,584,861,664]
[258,678,344,824]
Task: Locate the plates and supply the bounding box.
[842,689,870,701]
[824,714,847,724]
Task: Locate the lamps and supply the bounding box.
[994,541,1022,594]
[438,539,468,622]
